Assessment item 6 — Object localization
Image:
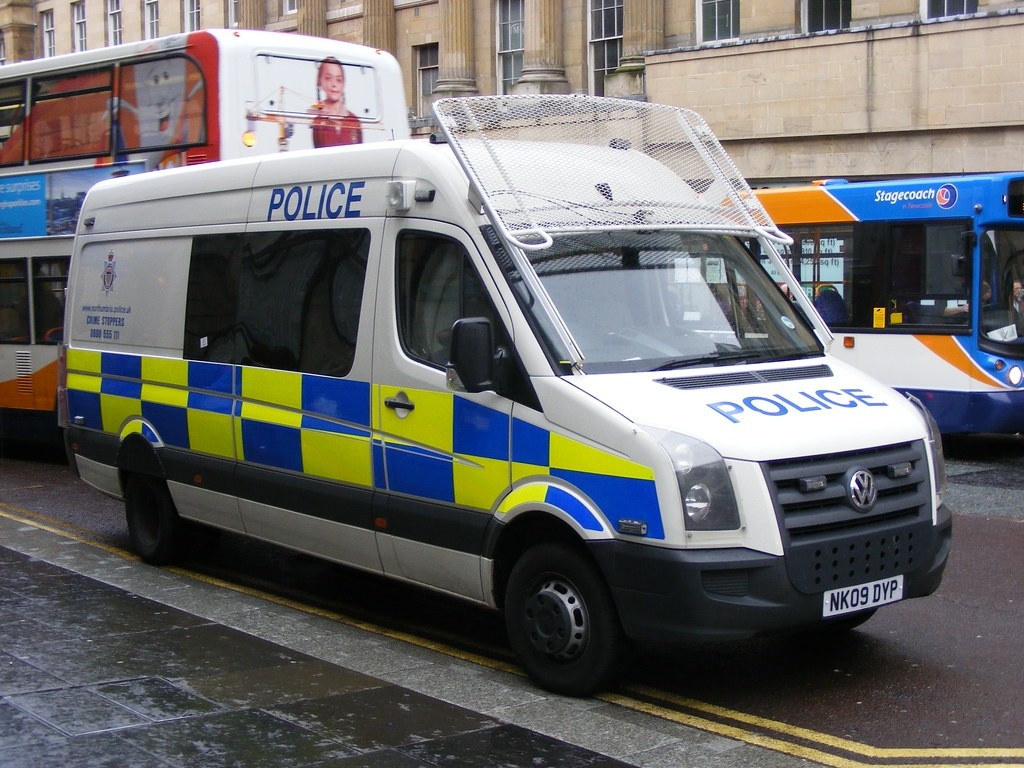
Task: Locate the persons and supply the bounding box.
[1010,280,1024,337]
[17,271,65,337]
[736,283,796,310]
[932,232,991,301]
[313,58,361,149]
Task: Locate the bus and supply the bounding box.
[0,26,413,466]
[652,168,1024,454]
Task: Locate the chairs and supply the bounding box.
[816,289,849,324]
[415,241,496,366]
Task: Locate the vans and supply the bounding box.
[53,89,956,700]
